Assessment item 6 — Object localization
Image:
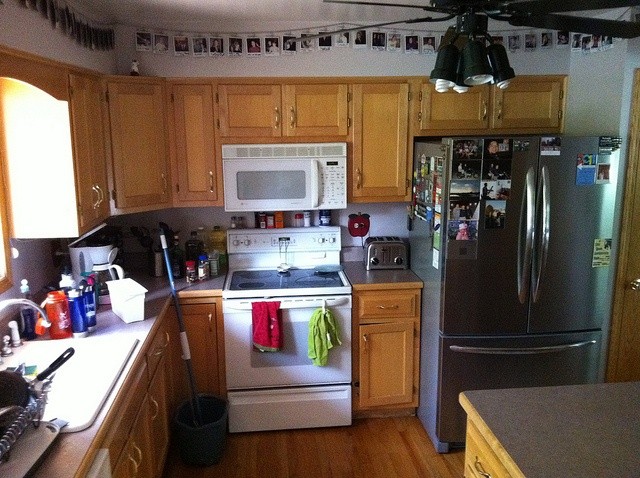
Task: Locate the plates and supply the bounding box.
[170,289,229,405]
[145,292,174,476]
[348,76,415,204]
[341,260,424,421]
[415,73,567,137]
[73,351,159,477]
[0,70,111,239]
[106,74,174,217]
[166,76,224,208]
[216,75,347,145]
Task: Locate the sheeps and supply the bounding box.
[0,295,51,356]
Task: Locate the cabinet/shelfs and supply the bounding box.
[87,276,99,308]
[237,216,245,229]
[67,289,88,338]
[197,255,210,282]
[79,284,97,332]
[230,216,237,229]
[260,214,266,229]
[35,290,73,339]
[208,253,220,277]
[295,214,304,227]
[304,211,311,227]
[186,261,195,284]
[169,235,185,279]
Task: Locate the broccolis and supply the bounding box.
[408,132,623,455]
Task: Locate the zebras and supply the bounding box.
[221,294,353,434]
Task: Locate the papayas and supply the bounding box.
[363,235,408,272]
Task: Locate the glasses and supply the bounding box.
[428,12,514,94]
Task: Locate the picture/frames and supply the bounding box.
[458,380,640,477]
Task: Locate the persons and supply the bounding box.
[424,38,434,50]
[267,41,272,52]
[210,40,220,52]
[270,43,279,51]
[453,204,472,220]
[458,162,472,177]
[408,37,416,49]
[486,204,501,227]
[249,41,260,52]
[482,182,494,200]
[456,223,470,241]
[155,37,166,49]
[489,163,504,179]
[232,41,241,52]
[355,32,364,44]
[338,33,348,43]
[389,35,398,48]
[487,141,498,155]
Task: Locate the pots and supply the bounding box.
[0,346,75,407]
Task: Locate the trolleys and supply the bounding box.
[324,0,640,39]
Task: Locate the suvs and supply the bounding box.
[172,393,229,467]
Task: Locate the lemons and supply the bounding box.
[92,248,124,305]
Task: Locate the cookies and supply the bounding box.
[221,142,348,212]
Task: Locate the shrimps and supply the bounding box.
[67,223,114,283]
